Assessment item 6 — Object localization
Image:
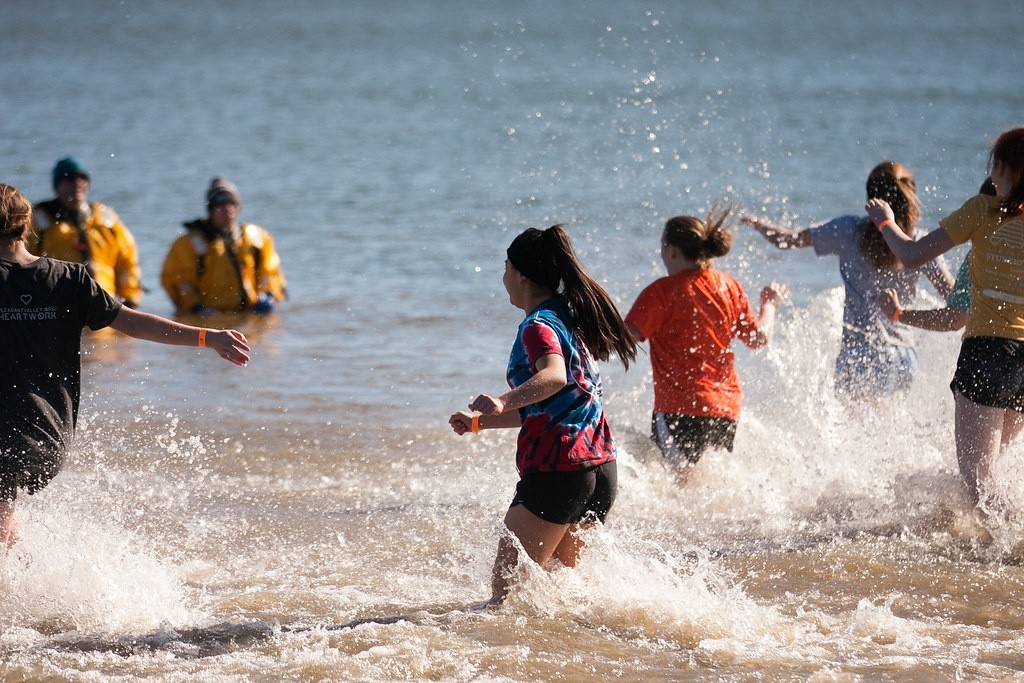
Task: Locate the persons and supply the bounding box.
[738,160,957,433]
[22,152,144,310]
[447,222,648,609]
[605,199,790,502]
[0,184,250,589]
[879,175,999,333]
[866,129,1024,510]
[159,172,291,313]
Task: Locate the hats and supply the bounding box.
[208,178,239,207]
[53,156,89,189]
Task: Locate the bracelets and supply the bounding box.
[198,327,207,347]
[893,308,903,321]
[471,414,481,435]
[879,219,896,232]
